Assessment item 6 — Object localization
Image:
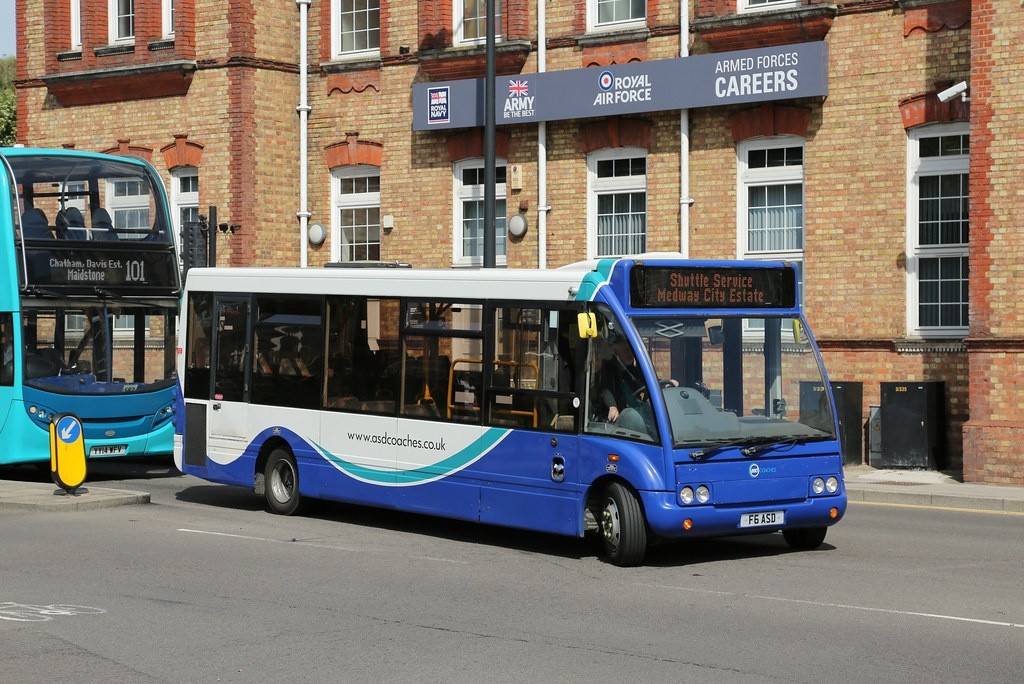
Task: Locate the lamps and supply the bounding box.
[309,223,327,245]
[383,215,394,233]
[509,214,528,238]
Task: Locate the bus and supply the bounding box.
[169,252,849,569]
[1,145,185,481]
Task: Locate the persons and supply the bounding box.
[279,336,334,378]
[594,335,679,423]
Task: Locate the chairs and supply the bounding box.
[262,347,597,430]
[17,206,55,240]
[34,349,63,380]
[55,207,88,240]
[92,207,119,241]
